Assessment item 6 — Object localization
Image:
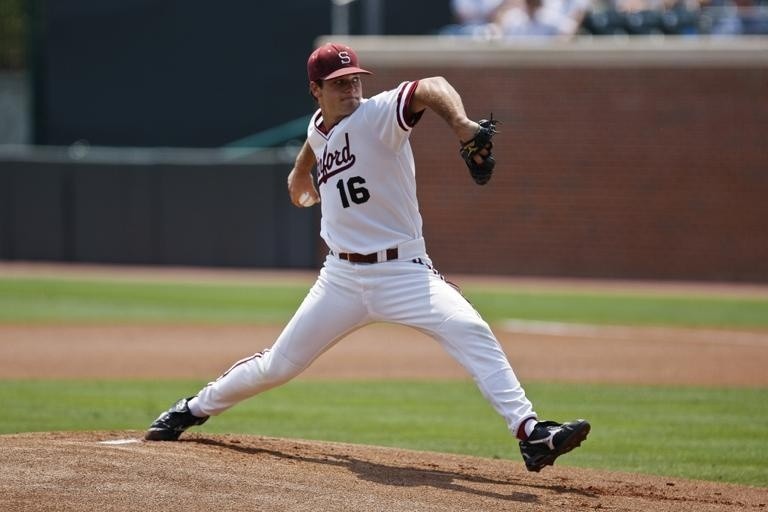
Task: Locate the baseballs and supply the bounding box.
[296,190,315,208]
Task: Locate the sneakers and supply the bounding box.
[518,414,592,474]
[143,392,213,444]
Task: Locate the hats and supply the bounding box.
[301,39,373,84]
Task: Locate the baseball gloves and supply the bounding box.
[459,113,501,185]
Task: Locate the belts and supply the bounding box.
[328,249,400,265]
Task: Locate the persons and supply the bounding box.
[140,40,591,474]
